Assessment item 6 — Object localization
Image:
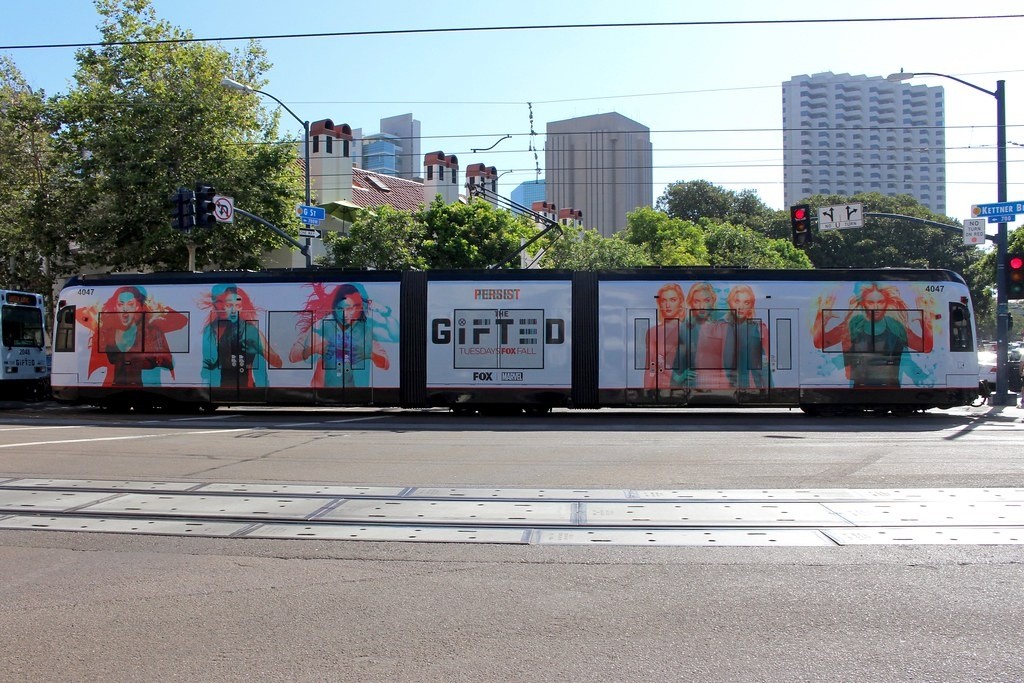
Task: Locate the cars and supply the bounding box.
[978,339,1024,395]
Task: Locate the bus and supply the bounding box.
[51,184,984,417]
[0,289,48,399]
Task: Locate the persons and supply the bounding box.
[698,283,774,388]
[645,283,685,387]
[197,284,282,386]
[289,282,399,387]
[811,281,937,388]
[705,282,730,321]
[76,286,188,386]
[670,282,717,388]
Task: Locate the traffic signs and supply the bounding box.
[818,205,841,230]
[839,202,863,229]
[297,227,323,240]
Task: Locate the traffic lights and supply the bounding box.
[194,182,218,230]
[791,203,813,248]
[170,187,195,232]
[1006,253,1024,300]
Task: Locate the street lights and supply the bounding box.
[886,72,1018,406]
[224,76,314,266]
